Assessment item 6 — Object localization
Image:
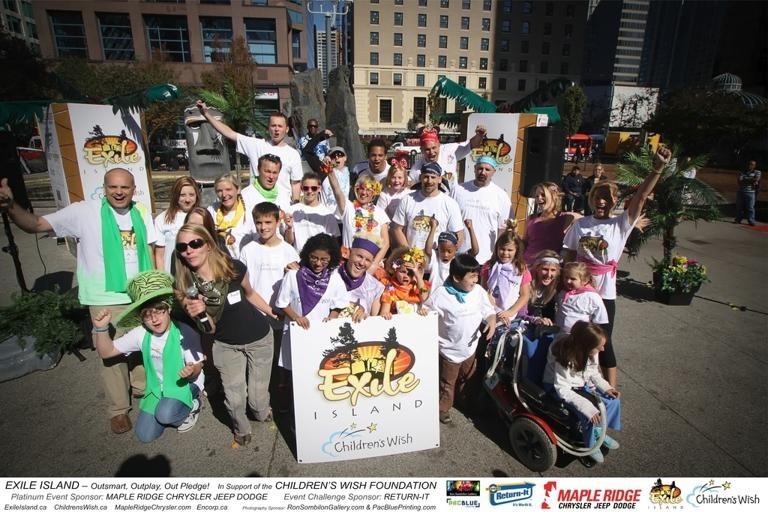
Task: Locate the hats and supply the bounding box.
[114,272,174,329]
[328,146,346,156]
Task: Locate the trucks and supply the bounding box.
[604,131,666,159]
[149,138,188,171]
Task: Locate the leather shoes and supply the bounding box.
[111,414,132,433]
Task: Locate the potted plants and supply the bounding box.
[612,144,725,307]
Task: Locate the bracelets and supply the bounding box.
[418,287,429,293]
[95,325,110,333]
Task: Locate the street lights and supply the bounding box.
[306,0,353,89]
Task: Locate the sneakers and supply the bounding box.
[440,412,451,423]
[177,399,201,432]
[589,441,604,463]
[204,376,219,397]
[604,436,619,449]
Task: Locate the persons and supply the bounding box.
[196,98,672,416]
[152,176,201,270]
[421,253,496,423]
[540,323,620,465]
[173,223,281,445]
[92,297,207,445]
[0,168,158,435]
[734,160,762,227]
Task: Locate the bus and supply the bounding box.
[391,131,461,156]
[564,133,592,162]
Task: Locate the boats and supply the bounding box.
[16,136,48,175]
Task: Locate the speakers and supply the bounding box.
[520,126,567,198]
[0,130,34,215]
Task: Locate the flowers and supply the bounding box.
[390,246,426,272]
[353,199,375,233]
[648,252,710,295]
[318,154,337,176]
[381,284,396,297]
[411,278,432,295]
[352,180,382,197]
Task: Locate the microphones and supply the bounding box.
[185,286,214,332]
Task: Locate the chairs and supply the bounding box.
[523,322,562,398]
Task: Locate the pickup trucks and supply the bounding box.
[389,142,421,156]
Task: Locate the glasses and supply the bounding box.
[302,186,317,191]
[308,255,330,264]
[330,152,344,159]
[175,238,206,252]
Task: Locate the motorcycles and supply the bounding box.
[480,319,609,471]
